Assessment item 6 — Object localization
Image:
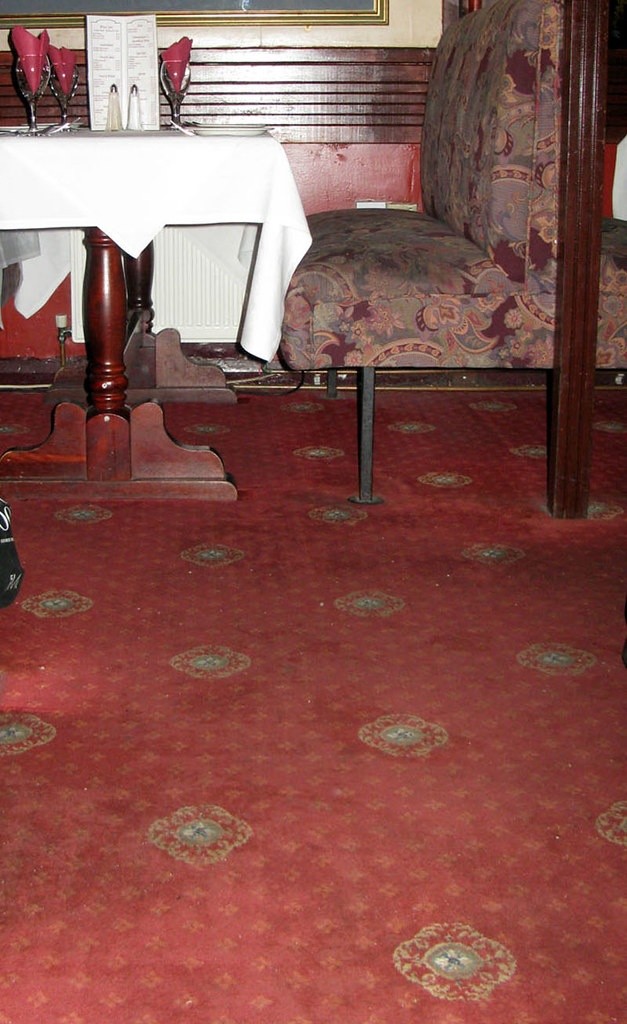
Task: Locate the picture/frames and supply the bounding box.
[0,0,390,30]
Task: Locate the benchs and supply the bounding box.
[594,218,627,392]
[279,1,567,505]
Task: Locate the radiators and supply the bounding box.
[71,226,251,344]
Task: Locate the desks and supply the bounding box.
[0,124,314,502]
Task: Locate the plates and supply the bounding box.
[0,123,82,132]
[195,124,273,135]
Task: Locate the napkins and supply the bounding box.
[11,26,50,95]
[160,36,193,94]
[47,45,76,95]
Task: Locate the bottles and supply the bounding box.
[108,84,120,131]
[127,85,141,131]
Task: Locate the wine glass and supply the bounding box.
[16,55,51,137]
[161,61,191,129]
[49,63,79,132]
